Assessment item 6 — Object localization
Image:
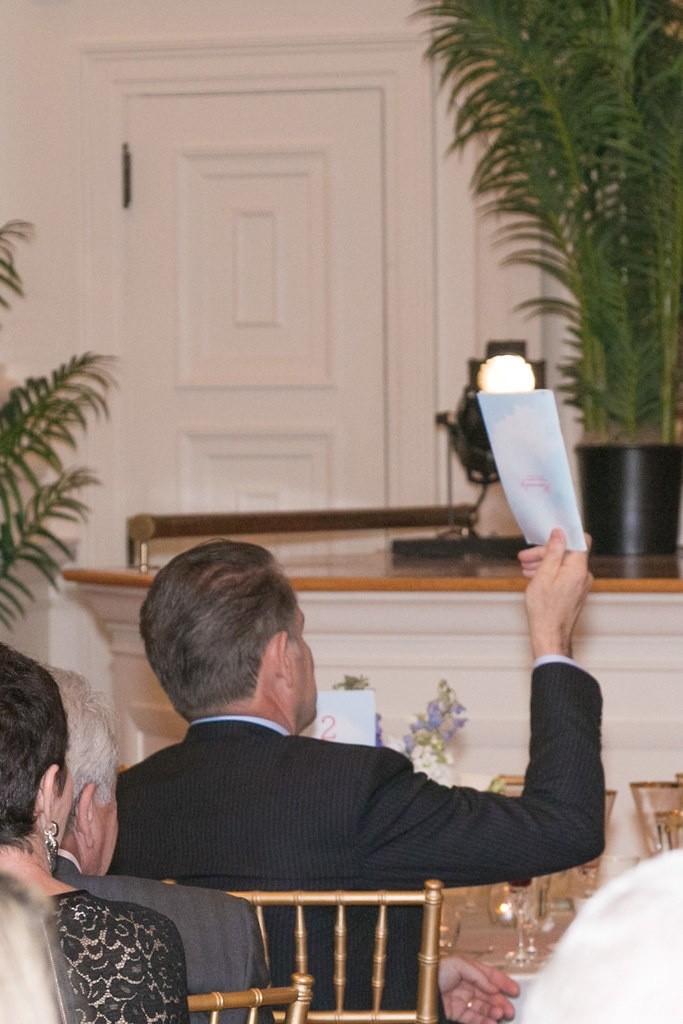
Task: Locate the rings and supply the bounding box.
[466,995,477,1009]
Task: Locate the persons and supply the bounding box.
[0,641,189,1024]
[33,660,269,1024]
[106,527,605,1024]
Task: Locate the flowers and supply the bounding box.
[333,675,467,788]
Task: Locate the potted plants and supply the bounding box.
[404,1,683,555]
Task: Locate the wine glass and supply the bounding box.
[499,877,539,976]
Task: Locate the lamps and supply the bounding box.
[454,337,547,475]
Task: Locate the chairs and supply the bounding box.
[162,877,443,1024]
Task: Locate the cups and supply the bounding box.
[488,883,519,927]
